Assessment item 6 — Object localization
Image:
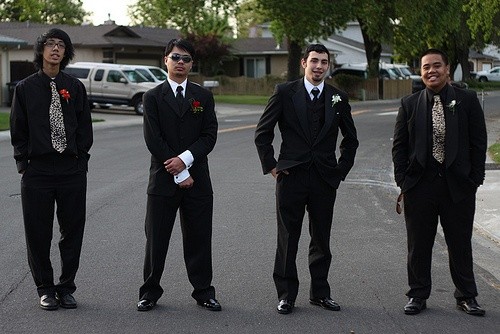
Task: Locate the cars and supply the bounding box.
[144,65,201,87]
[476,66,500,82]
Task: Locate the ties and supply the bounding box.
[432,95,445,164]
[176,86,185,115]
[311,89,320,103]
[49,81,68,154]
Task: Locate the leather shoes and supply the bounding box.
[197,299,221,311]
[40,294,77,310]
[276,299,295,314]
[309,296,340,311]
[456,298,485,316]
[137,300,155,311]
[403,297,427,315]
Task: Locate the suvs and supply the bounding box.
[329,65,405,80]
[388,63,425,93]
[62,63,158,116]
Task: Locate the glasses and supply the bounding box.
[396,193,404,214]
[167,53,192,63]
[44,40,66,49]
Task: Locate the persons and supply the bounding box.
[136,39,222,310]
[392,50,488,316]
[9,28,95,310]
[254,44,360,315]
